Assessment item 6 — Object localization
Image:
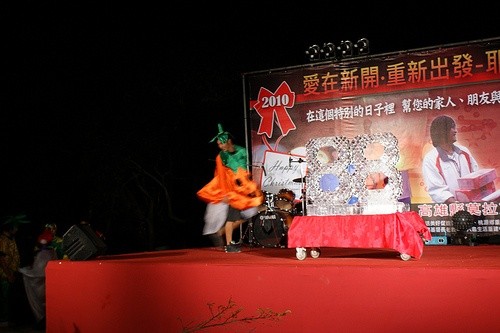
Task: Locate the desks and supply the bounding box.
[287,211,432,261]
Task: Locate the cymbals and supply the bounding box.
[293,178,305,182]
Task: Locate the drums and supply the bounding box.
[277,189,295,211]
[254,211,292,246]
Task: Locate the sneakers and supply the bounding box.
[224,245,241,253]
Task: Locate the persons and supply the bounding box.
[197,124,263,253]
[421,115,479,205]
[0,215,20,325]
